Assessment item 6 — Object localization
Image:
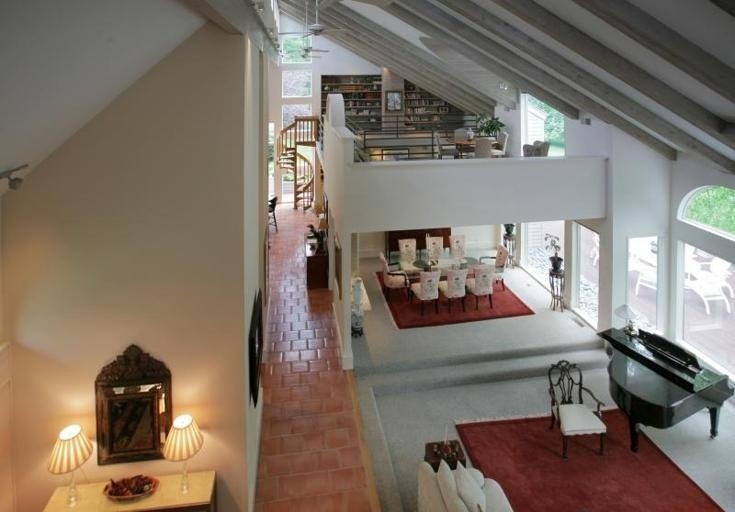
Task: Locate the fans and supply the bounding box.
[288,2,329,59]
[279,0,351,38]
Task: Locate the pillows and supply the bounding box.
[416,459,486,512]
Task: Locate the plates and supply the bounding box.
[102,476,159,500]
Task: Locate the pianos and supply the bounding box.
[597,327,735,452]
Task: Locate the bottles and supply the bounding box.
[466,128,474,141]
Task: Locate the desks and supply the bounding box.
[43,471,216,512]
[304,233,329,289]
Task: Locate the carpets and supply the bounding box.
[455,408,725,512]
[375,270,535,330]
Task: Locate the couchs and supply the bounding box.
[465,467,514,512]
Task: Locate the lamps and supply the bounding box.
[318,217,330,234]
[46,424,94,507]
[162,414,204,493]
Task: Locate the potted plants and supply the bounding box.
[544,233,563,272]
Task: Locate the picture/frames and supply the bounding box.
[385,90,403,111]
[381,148,409,161]
[94,343,173,465]
[249,287,264,409]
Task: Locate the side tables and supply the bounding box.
[424,439,466,473]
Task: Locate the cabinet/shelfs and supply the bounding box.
[405,78,464,129]
[321,75,382,131]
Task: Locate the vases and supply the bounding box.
[504,224,515,236]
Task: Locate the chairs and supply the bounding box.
[435,128,551,158]
[379,233,509,316]
[268,196,278,231]
[590,230,734,315]
[548,359,608,458]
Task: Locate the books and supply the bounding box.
[321,74,464,131]
